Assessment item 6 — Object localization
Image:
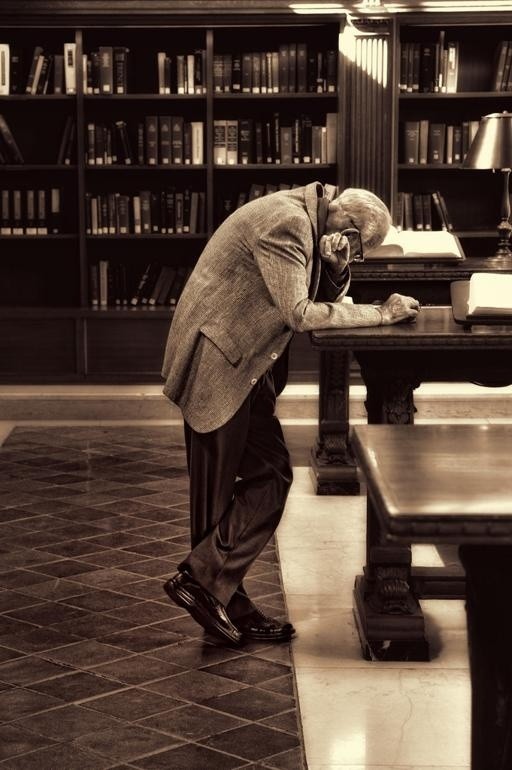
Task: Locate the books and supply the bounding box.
[214,43,336,93]
[465,272,510,319]
[213,113,336,165]
[223,183,337,221]
[84,115,204,165]
[1,119,26,164]
[158,49,206,94]
[399,30,512,231]
[2,188,61,235]
[91,259,192,306]
[55,117,76,165]
[86,191,206,235]
[83,46,129,94]
[1,43,76,95]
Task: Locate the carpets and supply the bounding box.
[271,463,472,770]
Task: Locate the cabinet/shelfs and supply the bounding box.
[1,3,511,382]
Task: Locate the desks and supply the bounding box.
[304,306,510,424]
[351,425,510,663]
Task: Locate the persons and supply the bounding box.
[159,177,424,647]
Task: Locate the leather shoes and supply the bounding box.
[224,607,296,641]
[164,567,242,646]
